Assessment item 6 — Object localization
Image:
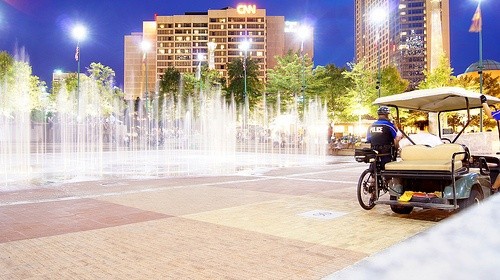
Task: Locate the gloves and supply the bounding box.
[480,95,488,103]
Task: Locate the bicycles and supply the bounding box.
[356,141,397,210]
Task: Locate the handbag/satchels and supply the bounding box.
[355,148,376,162]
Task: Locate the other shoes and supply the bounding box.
[490,187,498,194]
[369,199,372,205]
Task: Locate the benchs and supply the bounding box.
[384,144,465,172]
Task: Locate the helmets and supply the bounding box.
[377,107,390,114]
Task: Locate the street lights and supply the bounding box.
[239,38,251,98]
[140,41,153,116]
[370,7,385,98]
[71,23,86,111]
[297,25,310,115]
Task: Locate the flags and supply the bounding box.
[195,64,201,80]
[300,41,303,53]
[469,4,482,32]
[74,43,78,61]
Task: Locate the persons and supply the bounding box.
[398,120,444,148]
[332,133,355,155]
[481,95,500,120]
[366,106,404,204]
